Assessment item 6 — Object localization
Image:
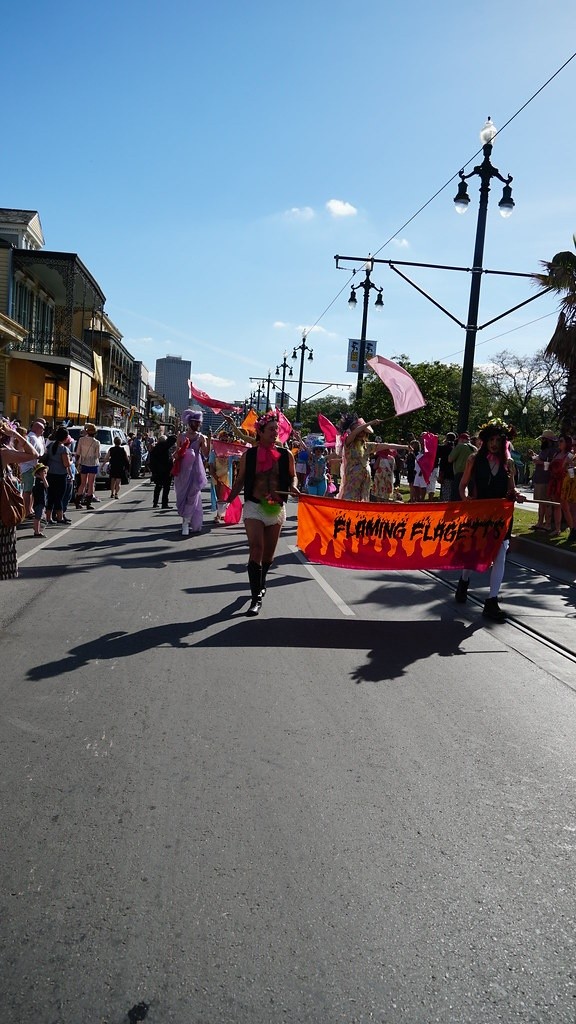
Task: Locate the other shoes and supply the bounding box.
[25,485,124,539]
[153,505,160,508]
[163,506,173,509]
[455,576,470,603]
[214,515,222,522]
[182,523,188,535]
[481,600,508,618]
[548,530,562,537]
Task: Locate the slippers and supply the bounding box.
[528,525,538,530]
[536,526,551,534]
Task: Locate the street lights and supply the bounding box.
[448,115,515,503]
[274,350,294,415]
[239,368,276,421]
[348,252,385,419]
[291,327,314,432]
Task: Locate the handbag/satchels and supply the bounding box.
[1,475,26,528]
[76,464,82,472]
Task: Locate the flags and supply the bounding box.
[318,413,339,443]
[242,409,259,433]
[277,409,292,442]
[191,387,242,413]
[367,355,426,415]
[213,441,249,456]
[220,485,242,524]
[417,433,439,485]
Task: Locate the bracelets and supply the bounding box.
[12,431,15,437]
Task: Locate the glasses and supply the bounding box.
[220,439,229,443]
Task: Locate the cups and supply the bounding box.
[543,462,550,471]
[568,466,574,478]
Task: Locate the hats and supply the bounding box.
[32,463,49,477]
[535,429,558,442]
[458,433,470,440]
[311,439,326,450]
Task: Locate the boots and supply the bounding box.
[262,560,275,597]
[247,560,263,616]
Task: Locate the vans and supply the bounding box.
[64,425,131,490]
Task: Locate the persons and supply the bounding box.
[436,432,481,502]
[528,430,576,540]
[456,419,526,620]
[226,416,300,616]
[0,418,101,580]
[284,419,438,501]
[129,410,284,535]
[100,437,130,499]
